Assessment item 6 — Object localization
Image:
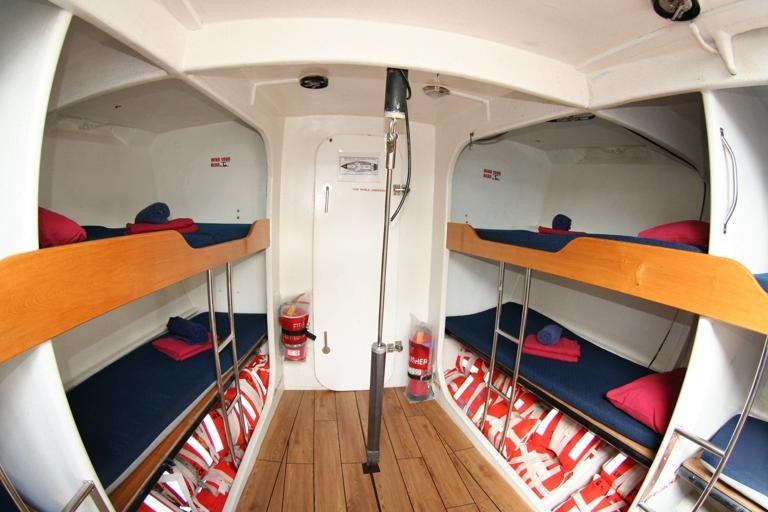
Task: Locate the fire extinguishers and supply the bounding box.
[278,292,309,363]
[405,322,433,402]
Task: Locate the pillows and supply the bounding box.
[638,217,707,246]
[604,364,684,434]
[32,203,88,250]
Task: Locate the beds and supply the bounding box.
[443,212,768,512]
[0,200,270,512]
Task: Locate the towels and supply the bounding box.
[519,326,581,363]
[120,205,201,230]
[156,314,217,359]
[537,215,579,236]
